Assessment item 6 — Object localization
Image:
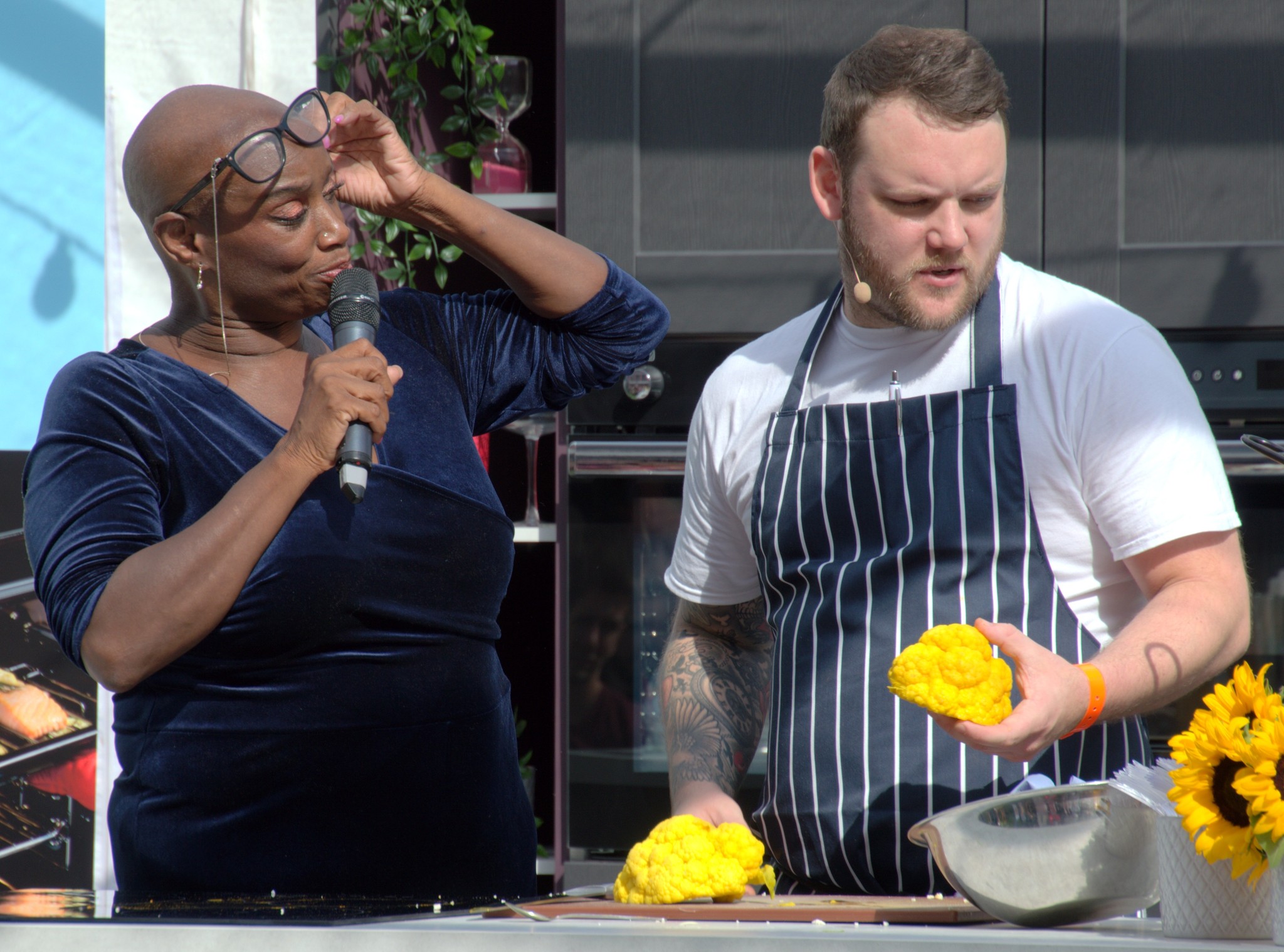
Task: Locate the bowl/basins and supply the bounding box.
[908,779,1162,927]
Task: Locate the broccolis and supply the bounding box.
[613,813,776,905]
[886,624,1013,727]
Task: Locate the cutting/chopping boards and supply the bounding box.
[485,893,1003,923]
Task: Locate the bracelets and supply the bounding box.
[1059,662,1106,741]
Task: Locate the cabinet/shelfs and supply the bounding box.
[317,0,1284,901]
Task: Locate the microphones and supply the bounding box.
[333,268,383,503]
[833,221,872,304]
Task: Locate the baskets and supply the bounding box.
[1158,817,1273,940]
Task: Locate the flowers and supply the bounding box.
[1168,661,1284,892]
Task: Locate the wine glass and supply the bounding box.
[504,409,558,528]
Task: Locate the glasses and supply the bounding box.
[169,87,331,212]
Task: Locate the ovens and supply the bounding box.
[1144,329,1284,744]
[556,337,775,892]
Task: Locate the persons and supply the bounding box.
[19,83,673,926]
[655,22,1252,899]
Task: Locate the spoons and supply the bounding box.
[505,902,666,922]
